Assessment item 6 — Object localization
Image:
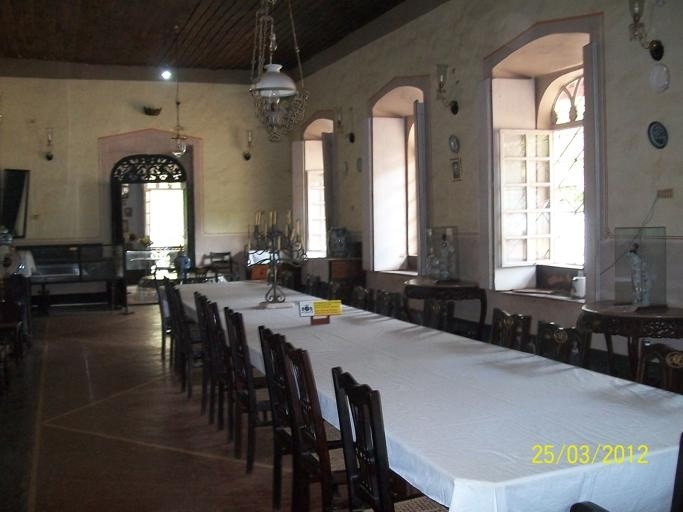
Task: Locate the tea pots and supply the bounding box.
[570,276,585,299]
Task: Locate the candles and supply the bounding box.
[247,209,301,252]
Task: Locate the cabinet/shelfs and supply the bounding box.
[16,241,129,314]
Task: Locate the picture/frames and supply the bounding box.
[122,220,129,232]
[449,158,463,181]
[125,208,132,217]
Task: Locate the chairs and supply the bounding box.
[210,251,241,281]
[329,364,449,511]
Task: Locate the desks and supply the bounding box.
[126,250,185,270]
[302,257,361,285]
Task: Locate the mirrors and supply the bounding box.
[0,169,31,239]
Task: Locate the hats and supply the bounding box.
[1,233,12,244]
[0,225,8,234]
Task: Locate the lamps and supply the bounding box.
[45,127,53,161]
[437,64,459,115]
[335,106,354,143]
[247,1,310,142]
[242,130,254,160]
[628,1,664,61]
[170,24,188,159]
